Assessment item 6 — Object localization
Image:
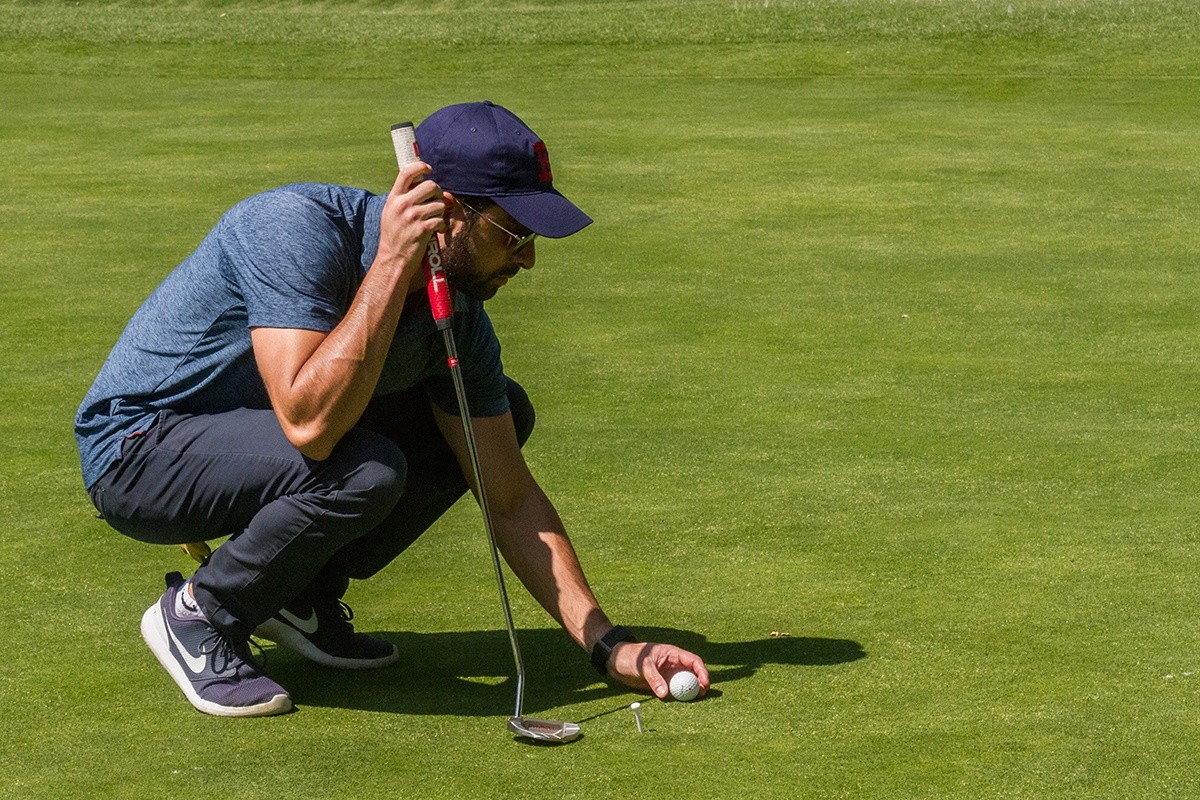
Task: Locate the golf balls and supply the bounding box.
[669,670,701,702]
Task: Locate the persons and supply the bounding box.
[75,102,709,717]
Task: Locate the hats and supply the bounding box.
[415,100,593,239]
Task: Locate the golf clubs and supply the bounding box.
[387,115,587,745]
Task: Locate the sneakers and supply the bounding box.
[251,597,399,669]
[141,571,291,717]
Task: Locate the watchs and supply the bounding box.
[590,624,636,676]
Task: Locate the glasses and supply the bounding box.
[458,200,539,250]
